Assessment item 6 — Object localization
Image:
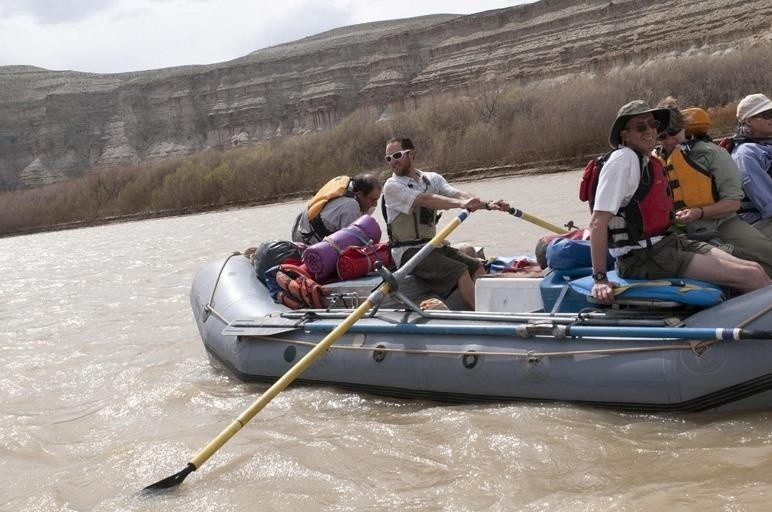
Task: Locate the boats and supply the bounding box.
[188,245,771,417]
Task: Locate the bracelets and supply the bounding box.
[485,199,493,210]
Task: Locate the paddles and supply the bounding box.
[142,209,471,490]
[222,319,771,340]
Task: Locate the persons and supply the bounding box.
[590,93,772,304]
[381,137,486,311]
[291,173,382,246]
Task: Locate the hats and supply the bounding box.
[607,99,672,150]
[736,94,772,123]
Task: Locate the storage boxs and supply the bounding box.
[474,276,546,311]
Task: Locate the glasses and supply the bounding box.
[625,120,681,141]
[751,109,772,120]
[384,150,411,163]
